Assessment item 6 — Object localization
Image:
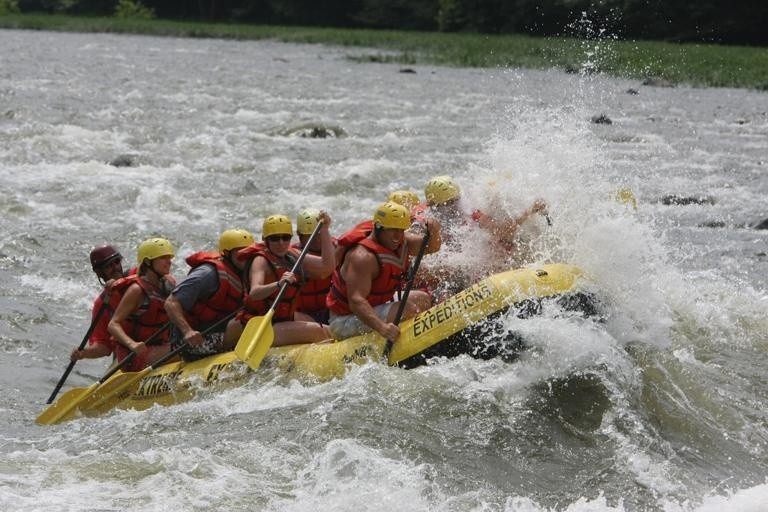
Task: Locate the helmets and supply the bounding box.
[136,238,175,264]
[262,214,293,238]
[373,201,410,230]
[219,229,253,256]
[388,190,421,207]
[425,176,461,206]
[91,245,123,273]
[297,208,322,235]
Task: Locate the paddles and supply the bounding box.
[33,322,170,426]
[234,217,324,372]
[79,302,244,417]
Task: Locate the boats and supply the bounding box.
[97,262,597,416]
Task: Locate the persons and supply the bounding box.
[69,174,550,370]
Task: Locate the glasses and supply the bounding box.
[270,233,291,242]
